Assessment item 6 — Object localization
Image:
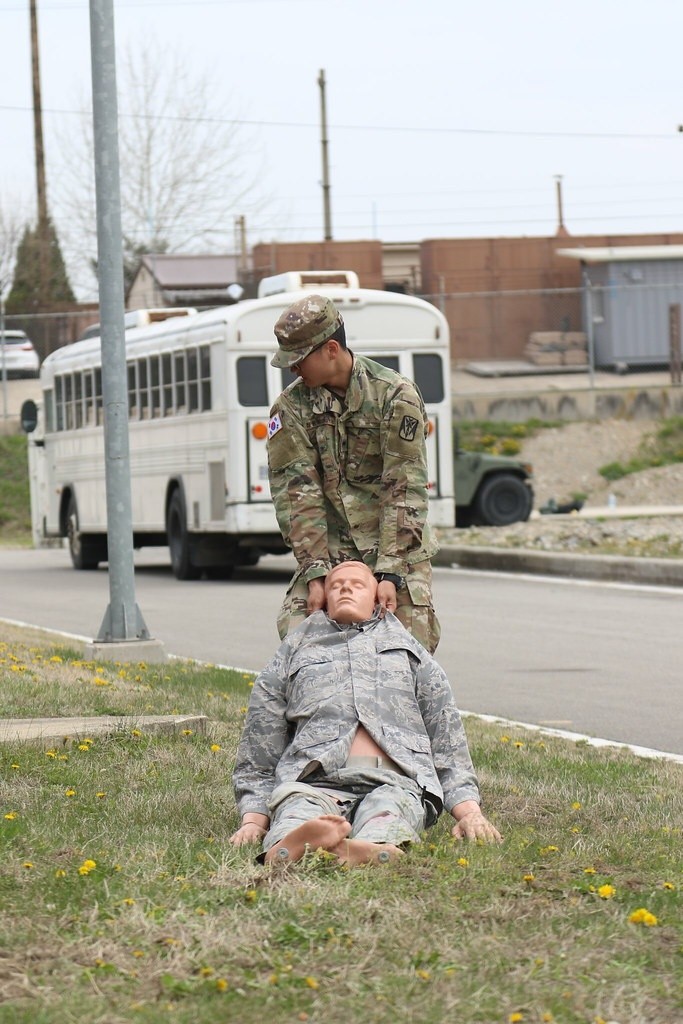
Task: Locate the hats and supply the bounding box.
[270,294,344,369]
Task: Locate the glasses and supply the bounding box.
[295,338,338,370]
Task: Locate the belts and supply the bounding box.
[340,756,406,776]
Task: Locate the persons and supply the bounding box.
[230,560,502,868]
[267,295,440,655]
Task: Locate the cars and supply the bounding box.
[449,444,538,527]
[0,329,41,378]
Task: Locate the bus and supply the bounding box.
[20,269,453,582]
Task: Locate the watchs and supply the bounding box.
[378,572,406,592]
